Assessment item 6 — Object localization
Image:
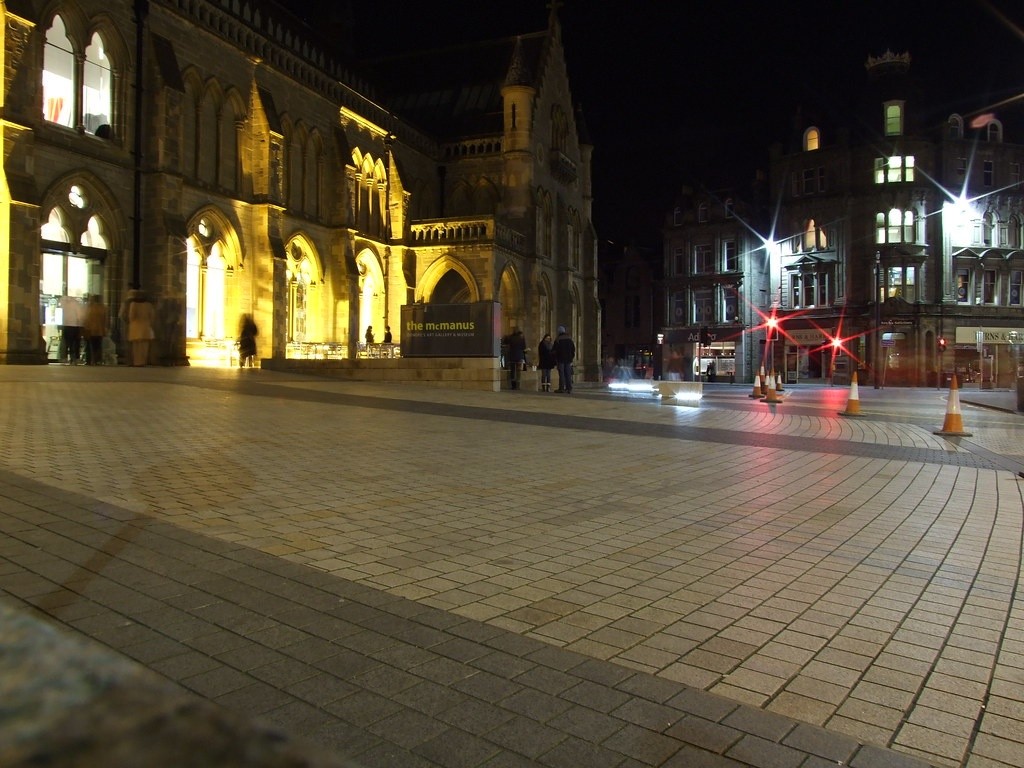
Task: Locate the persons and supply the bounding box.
[706,361,716,382]
[63,296,108,366]
[539,334,554,391]
[553,326,575,393]
[238,313,257,367]
[382,326,392,356]
[119,290,156,366]
[365,326,375,345]
[509,332,524,389]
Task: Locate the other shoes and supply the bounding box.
[568,386,572,394]
[554,389,565,393]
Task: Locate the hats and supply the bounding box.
[558,326,565,332]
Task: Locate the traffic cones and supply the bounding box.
[931,375,973,438]
[757,362,770,395]
[836,370,868,417]
[776,371,785,391]
[759,367,783,404]
[749,369,765,398]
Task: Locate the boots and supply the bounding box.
[546,383,550,392]
[541,383,546,392]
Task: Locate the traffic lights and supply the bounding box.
[938,337,946,352]
[765,316,779,342]
[830,338,841,357]
